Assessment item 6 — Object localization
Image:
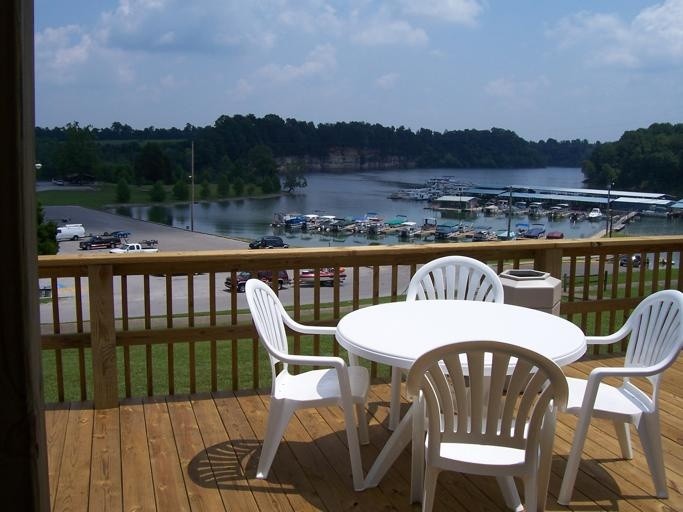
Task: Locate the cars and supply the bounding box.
[248,235,288,249]
[55,180,64,186]
[620,255,640,267]
[155,272,200,276]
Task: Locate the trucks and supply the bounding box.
[55,223,85,241]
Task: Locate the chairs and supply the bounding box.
[244,277,370,494]
[389,256,503,430]
[552,290,683,506]
[406,341,568,512]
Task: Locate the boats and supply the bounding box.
[298,267,346,282]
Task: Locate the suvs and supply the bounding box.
[225,269,288,293]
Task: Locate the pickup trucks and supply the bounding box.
[79,235,120,249]
[108,243,158,255]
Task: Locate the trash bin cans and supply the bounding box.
[497,269,562,393]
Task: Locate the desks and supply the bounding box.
[334,299,587,512]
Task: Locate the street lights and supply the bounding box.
[186,141,194,232]
